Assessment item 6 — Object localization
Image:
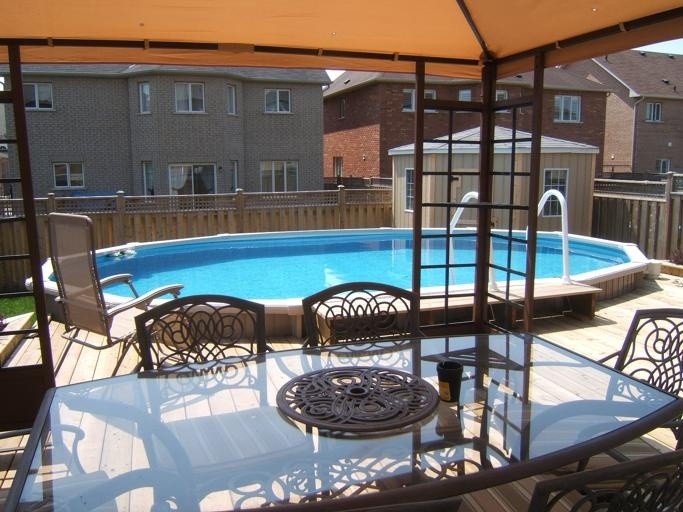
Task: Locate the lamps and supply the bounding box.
[43,204,208,379]
[295,279,470,512]
[131,287,321,512]
[452,301,683,512]
[522,448,682,511]
[1,419,127,512]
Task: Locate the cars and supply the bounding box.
[437,359,464,401]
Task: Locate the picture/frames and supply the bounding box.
[0,327,683,510]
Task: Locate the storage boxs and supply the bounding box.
[308,277,608,345]
